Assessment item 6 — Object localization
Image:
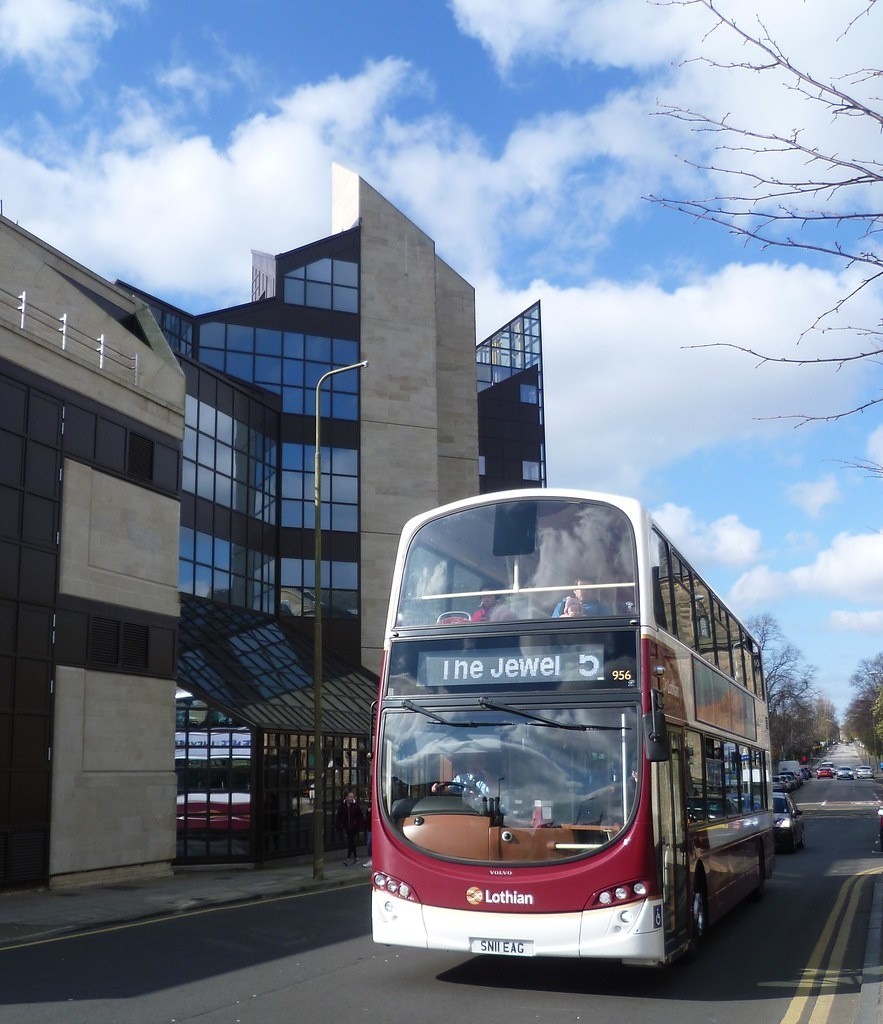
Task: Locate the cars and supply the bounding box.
[772,775,788,792]
[856,766,874,778]
[777,776,791,791]
[772,792,805,852]
[784,774,795,791]
[800,768,809,780]
[836,766,854,780]
[800,765,812,777]
[780,771,801,789]
[816,739,851,748]
[820,762,837,775]
[817,767,834,779]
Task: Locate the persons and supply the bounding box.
[621,759,638,819]
[549,576,611,618]
[472,584,516,625]
[361,799,372,866]
[557,597,587,620]
[429,749,505,814]
[335,792,364,867]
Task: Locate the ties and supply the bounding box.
[466,778,477,795]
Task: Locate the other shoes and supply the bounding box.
[353,858,360,865]
[343,858,353,866]
[362,859,372,867]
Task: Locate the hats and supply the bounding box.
[563,596,583,616]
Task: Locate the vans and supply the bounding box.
[778,760,804,785]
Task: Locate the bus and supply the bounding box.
[372,487,777,967]
[175,690,254,837]
